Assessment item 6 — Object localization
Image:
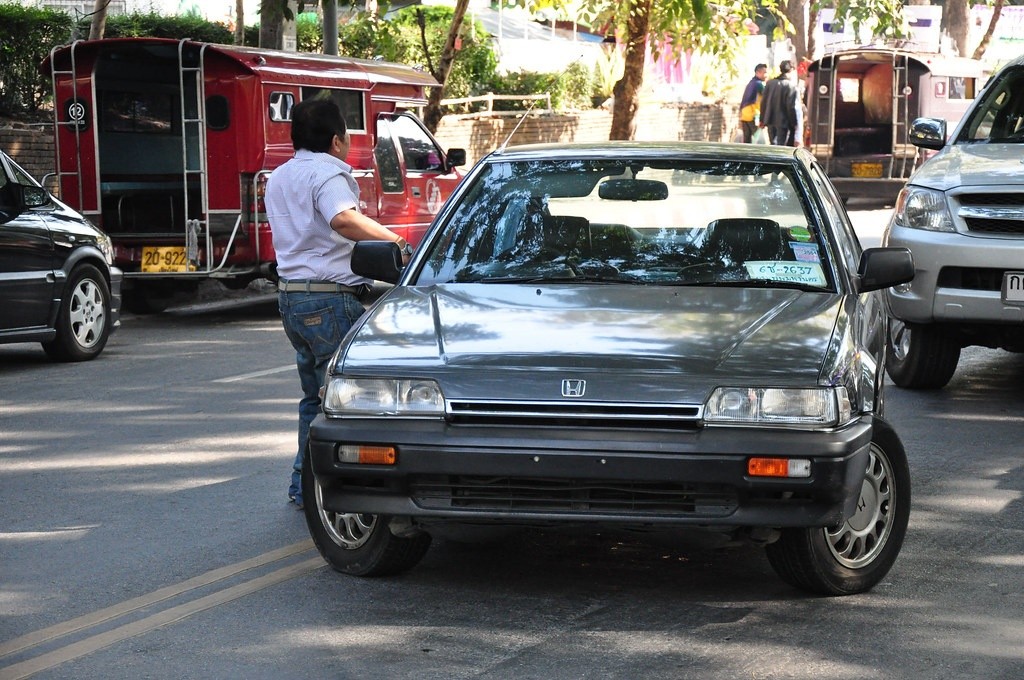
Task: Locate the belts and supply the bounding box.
[277,279,359,292]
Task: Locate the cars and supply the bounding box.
[879,54,1024,391]
[0,149,117,363]
[297,138,914,595]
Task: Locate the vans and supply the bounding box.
[36,32,467,318]
[799,42,935,210]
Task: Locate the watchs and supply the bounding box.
[401,243,413,255]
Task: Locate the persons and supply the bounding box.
[759,60,800,146]
[264,101,413,508]
[802,77,809,122]
[740,64,768,143]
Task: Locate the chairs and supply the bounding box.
[531,216,591,258]
[702,218,783,261]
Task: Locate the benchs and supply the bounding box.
[101,173,200,229]
[834,126,886,156]
